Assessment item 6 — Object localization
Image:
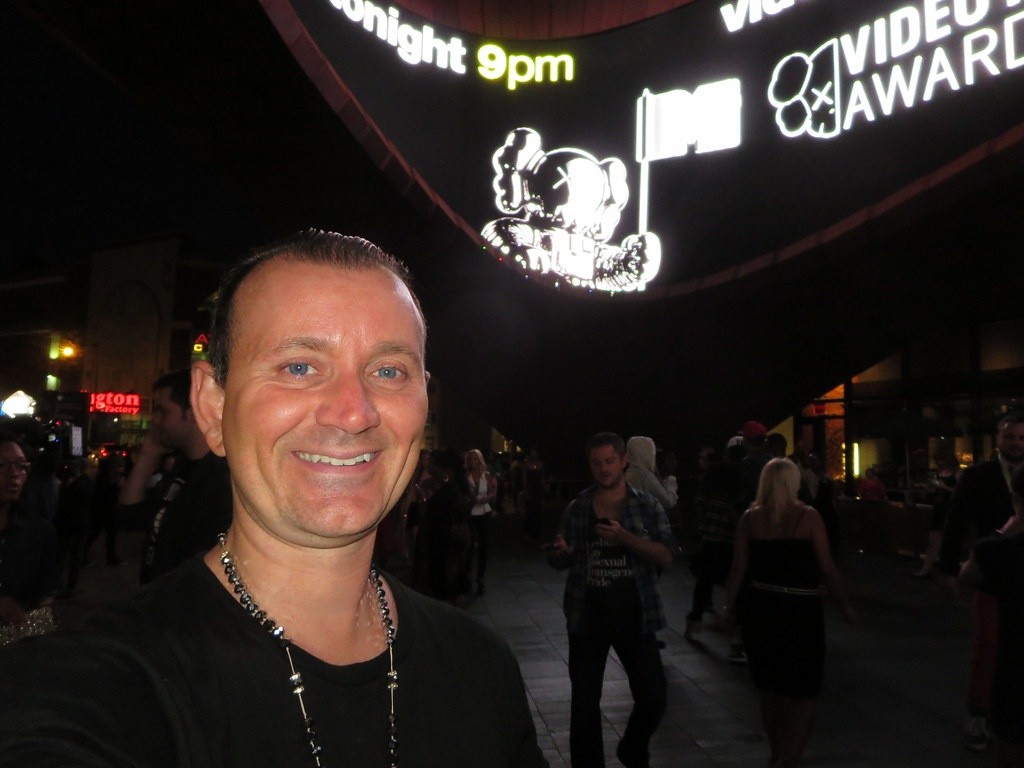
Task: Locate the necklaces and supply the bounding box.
[216,533,399,768]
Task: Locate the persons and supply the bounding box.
[856,411,1024,768]
[1,229,549,768]
[548,432,676,768]
[677,418,845,768]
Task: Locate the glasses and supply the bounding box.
[0,461,31,474]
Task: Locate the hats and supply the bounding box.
[727,436,744,448]
[736,421,767,440]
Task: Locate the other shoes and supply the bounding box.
[911,570,934,581]
[962,715,988,752]
[477,580,485,595]
[616,748,650,768]
[106,558,123,566]
[686,611,702,624]
[727,643,749,663]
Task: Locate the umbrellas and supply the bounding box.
[965,410,1006,449]
[846,402,964,488]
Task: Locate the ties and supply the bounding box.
[1008,464,1016,479]
